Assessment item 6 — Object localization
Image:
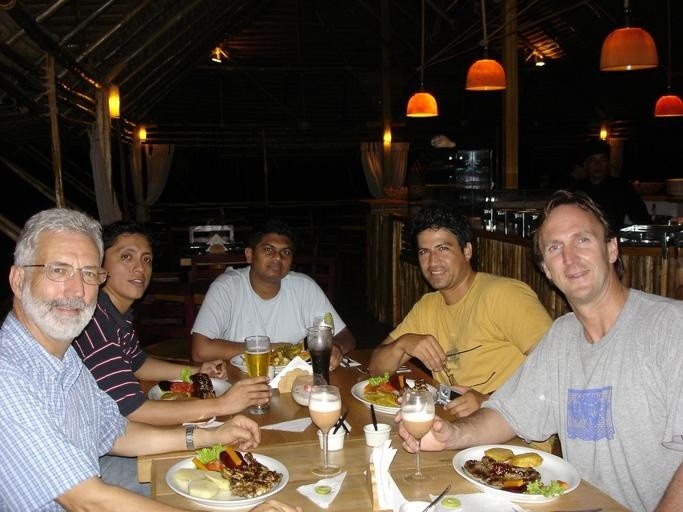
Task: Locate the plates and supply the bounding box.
[350,375,440,414]
[226,350,311,372]
[452,444,581,502]
[162,448,291,508]
[148,377,232,400]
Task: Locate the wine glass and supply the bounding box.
[306,384,342,477]
[397,390,436,484]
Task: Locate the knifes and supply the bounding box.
[340,361,345,369]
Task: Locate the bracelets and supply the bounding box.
[185,423,195,451]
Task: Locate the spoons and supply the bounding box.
[425,482,452,510]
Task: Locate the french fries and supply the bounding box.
[503,478,523,488]
[192,457,208,471]
[226,447,242,465]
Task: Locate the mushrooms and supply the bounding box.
[174,468,231,498]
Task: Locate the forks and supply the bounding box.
[343,356,355,365]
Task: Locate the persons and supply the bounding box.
[561,141,653,231]
[392,189,683,512]
[188,219,357,372]
[0,207,260,512]
[79,218,269,425]
[368,211,560,456]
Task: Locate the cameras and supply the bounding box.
[436,384,463,406]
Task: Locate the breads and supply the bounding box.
[485,448,514,468]
[512,453,543,468]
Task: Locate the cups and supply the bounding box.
[363,422,392,445]
[243,334,274,415]
[307,326,333,384]
[315,426,347,452]
[397,499,439,512]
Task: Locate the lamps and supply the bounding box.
[404,1,441,121]
[597,0,660,72]
[103,82,123,123]
[458,1,510,95]
[653,0,683,119]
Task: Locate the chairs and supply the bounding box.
[119,221,341,342]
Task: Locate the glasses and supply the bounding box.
[23,260,108,286]
